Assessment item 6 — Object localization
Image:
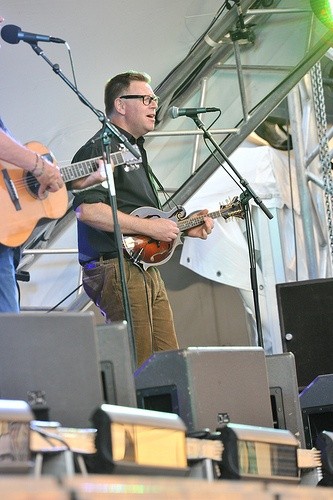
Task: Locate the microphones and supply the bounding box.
[168,106,220,118]
[1,24,66,44]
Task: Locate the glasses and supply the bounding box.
[119,94,159,106]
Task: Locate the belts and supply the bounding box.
[95,251,132,262]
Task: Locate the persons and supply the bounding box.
[0,118,116,316]
[72,71,215,371]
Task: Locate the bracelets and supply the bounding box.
[30,151,44,177]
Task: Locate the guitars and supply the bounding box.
[121,196,246,271]
[0,141,142,248]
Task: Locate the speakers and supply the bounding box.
[0,312,102,429]
[265,352,306,450]
[97,319,137,408]
[276,277,333,450]
[133,348,274,433]
